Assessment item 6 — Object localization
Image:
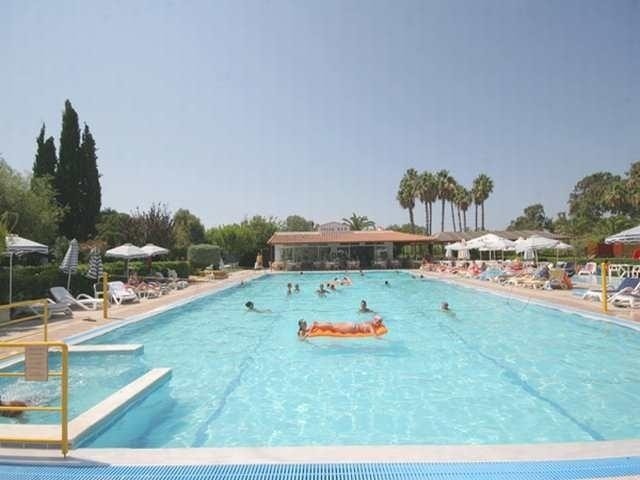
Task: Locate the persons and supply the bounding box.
[358,299,371,312]
[125,271,159,290]
[441,302,449,309]
[245,278,351,313]
[309,315,382,335]
[298,318,313,337]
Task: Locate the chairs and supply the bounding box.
[435,260,640,310]
[9,280,190,322]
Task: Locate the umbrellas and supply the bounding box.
[59,237,79,292]
[605,226,640,247]
[139,243,170,268]
[444,232,573,267]
[105,242,151,280]
[85,247,104,285]
[0,233,49,304]
